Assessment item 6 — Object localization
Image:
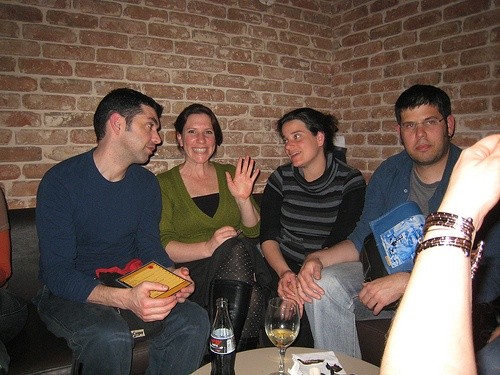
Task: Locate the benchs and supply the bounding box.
[0,193,500,375]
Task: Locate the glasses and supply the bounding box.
[399,114,450,131]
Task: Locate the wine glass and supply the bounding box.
[264,296,300,375]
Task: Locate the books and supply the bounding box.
[114,260,193,300]
[369,201,426,274]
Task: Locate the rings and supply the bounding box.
[280,296,285,300]
[280,314,285,315]
[291,304,295,307]
[289,307,294,311]
[280,308,285,311]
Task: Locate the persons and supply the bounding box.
[296,85,500,368]
[377,134,500,374]
[156,103,262,364]
[33,87,211,375]
[258,107,367,355]
[0,183,14,375]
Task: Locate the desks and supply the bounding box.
[189,346,381,375]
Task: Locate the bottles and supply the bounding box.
[209,297,236,375]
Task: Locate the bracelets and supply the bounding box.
[410,210,486,278]
[280,269,293,279]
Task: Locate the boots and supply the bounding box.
[213,279,253,351]
